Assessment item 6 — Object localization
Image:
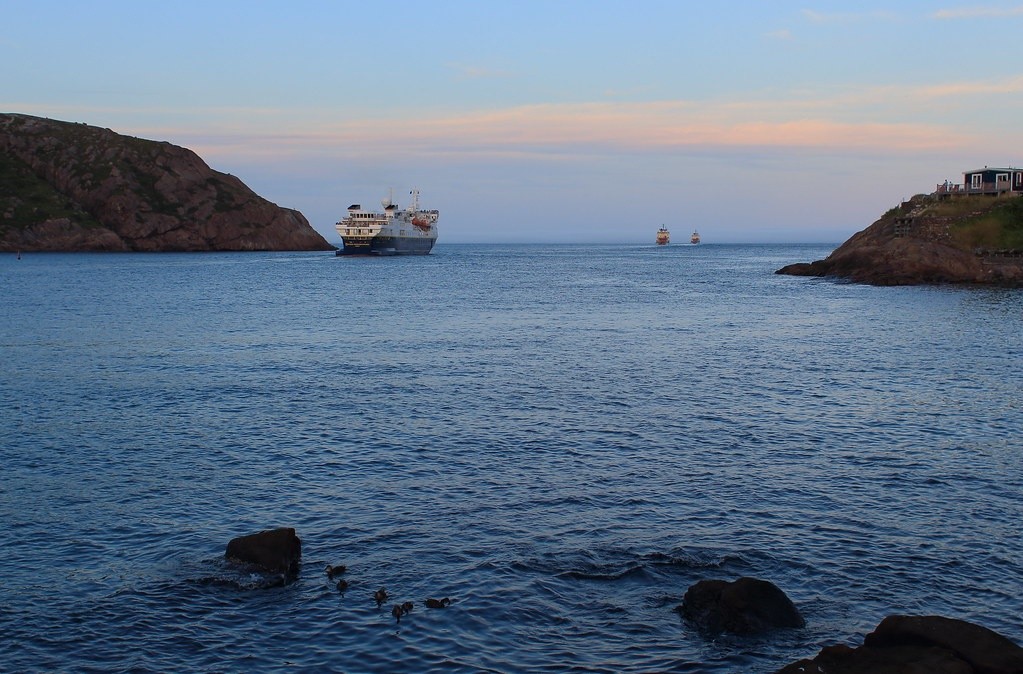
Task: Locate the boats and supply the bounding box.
[656,225,671,246]
[690,229,700,244]
[335,185,439,255]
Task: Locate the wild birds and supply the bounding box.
[422,597,449,608]
[392,602,413,624]
[336,580,350,598]
[375,588,386,606]
[324,565,345,576]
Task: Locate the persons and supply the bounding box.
[949,182,952,191]
[945,179,948,191]
